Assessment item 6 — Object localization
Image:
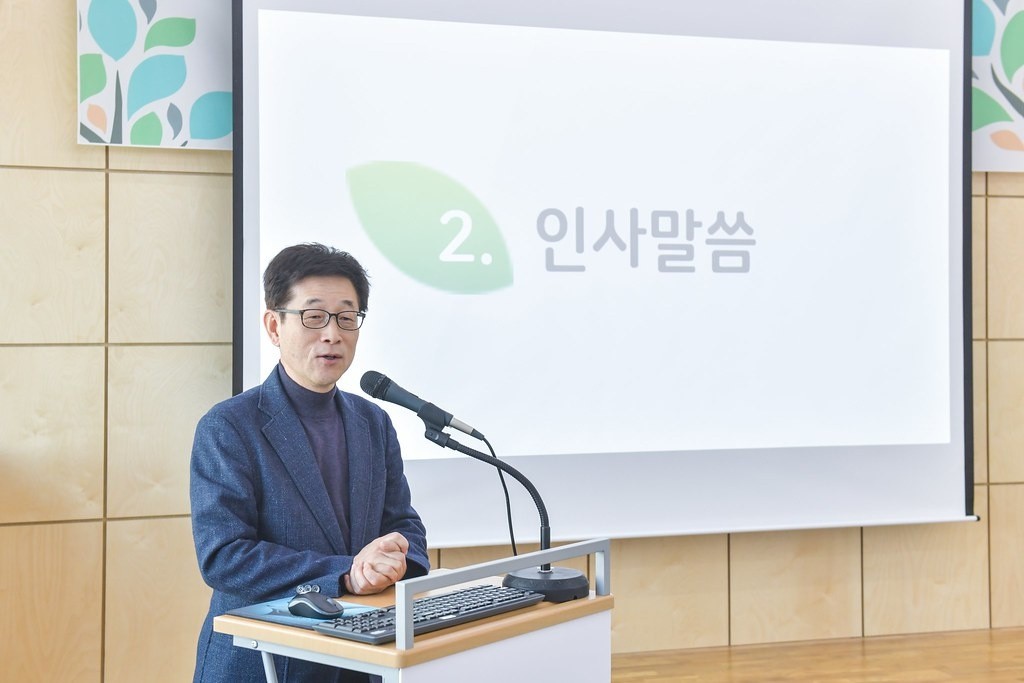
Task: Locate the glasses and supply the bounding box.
[273,308,366,331]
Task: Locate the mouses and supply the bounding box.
[288,592,344,620]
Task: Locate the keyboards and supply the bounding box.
[312,584,545,645]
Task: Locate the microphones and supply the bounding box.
[360,370,484,441]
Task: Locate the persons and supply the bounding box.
[190,242,431,683]
